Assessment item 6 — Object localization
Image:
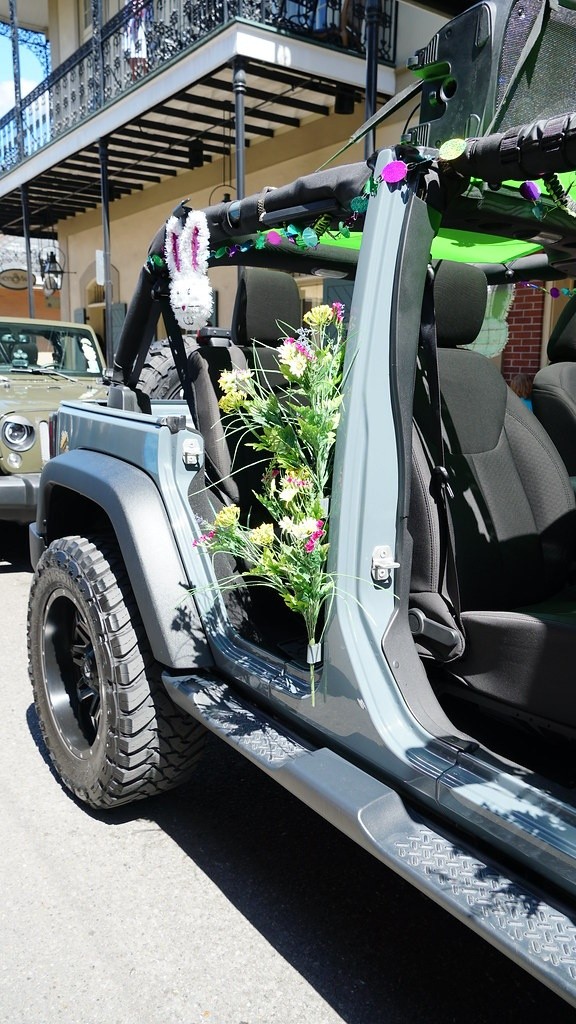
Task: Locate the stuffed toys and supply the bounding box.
[165,207,215,331]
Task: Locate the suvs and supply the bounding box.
[0,317,117,526]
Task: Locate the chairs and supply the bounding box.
[532,294,576,500]
[407,260,576,740]
[12,342,38,366]
[187,269,330,570]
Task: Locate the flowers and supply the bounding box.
[178,302,401,707]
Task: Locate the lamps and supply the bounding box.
[38,206,65,291]
[335,85,354,115]
[209,100,236,207]
[189,139,203,168]
